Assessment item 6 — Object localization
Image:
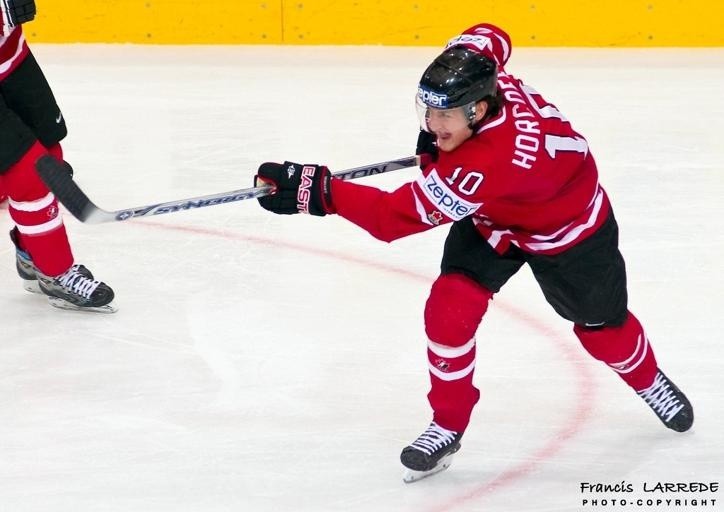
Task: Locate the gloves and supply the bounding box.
[416,130,439,171]
[254,161,333,216]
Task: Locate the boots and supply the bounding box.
[16,247,114,307]
[636,367,694,432]
[400,422,464,472]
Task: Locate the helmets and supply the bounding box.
[416,44,498,134]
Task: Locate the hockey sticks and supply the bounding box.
[33,153,434,227]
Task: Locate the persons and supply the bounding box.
[250,22,696,474]
[0,0,118,314]
